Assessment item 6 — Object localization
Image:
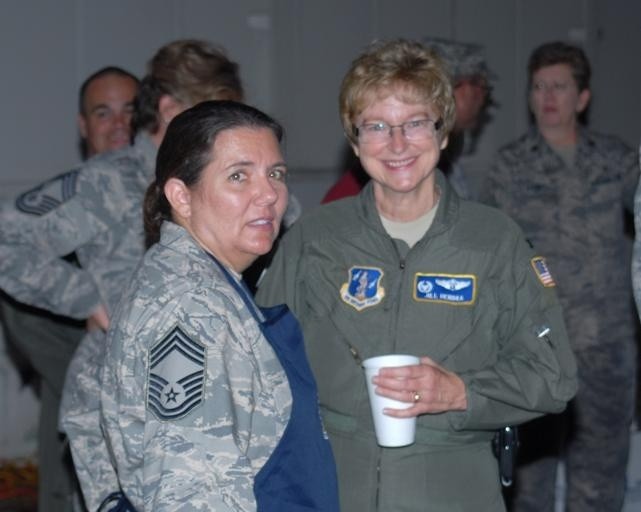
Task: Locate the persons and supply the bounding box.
[473,40,640,512]
[95,99,343,512]
[0,65,143,511]
[0,39,300,512]
[253,37,582,512]
[418,29,504,136]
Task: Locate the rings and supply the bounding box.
[414,392,421,404]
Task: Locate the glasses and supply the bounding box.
[352,119,444,144]
[455,80,496,97]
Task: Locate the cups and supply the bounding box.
[362,354,422,449]
